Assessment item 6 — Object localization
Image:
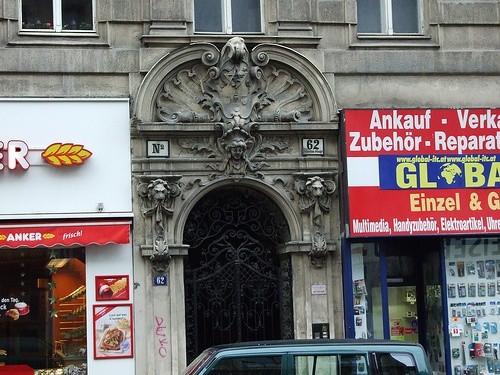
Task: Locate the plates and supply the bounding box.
[20,310,30,316]
[98,328,125,353]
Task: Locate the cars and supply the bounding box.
[174,337,433,375]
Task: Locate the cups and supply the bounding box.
[15,302,30,314]
